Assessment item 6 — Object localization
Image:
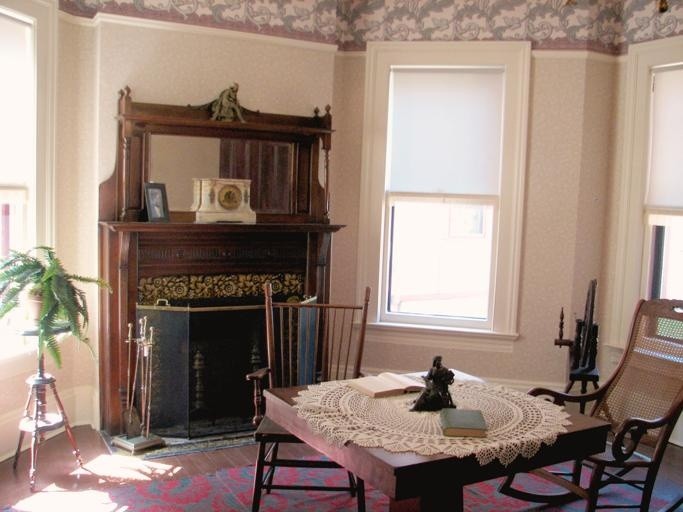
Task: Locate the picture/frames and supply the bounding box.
[143,183,170,222]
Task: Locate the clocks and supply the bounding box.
[194,176,258,226]
[187,176,202,212]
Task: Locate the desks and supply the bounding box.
[262,369,612,510]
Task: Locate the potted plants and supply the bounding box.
[0,241,119,371]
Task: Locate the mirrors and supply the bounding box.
[131,118,313,219]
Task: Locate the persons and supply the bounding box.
[422,355,455,399]
[211,83,248,124]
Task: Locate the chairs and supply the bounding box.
[245,280,373,511]
[497,296,683,512]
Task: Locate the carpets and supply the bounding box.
[110,453,683,512]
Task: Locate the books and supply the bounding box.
[441,408,488,438]
[345,372,426,399]
